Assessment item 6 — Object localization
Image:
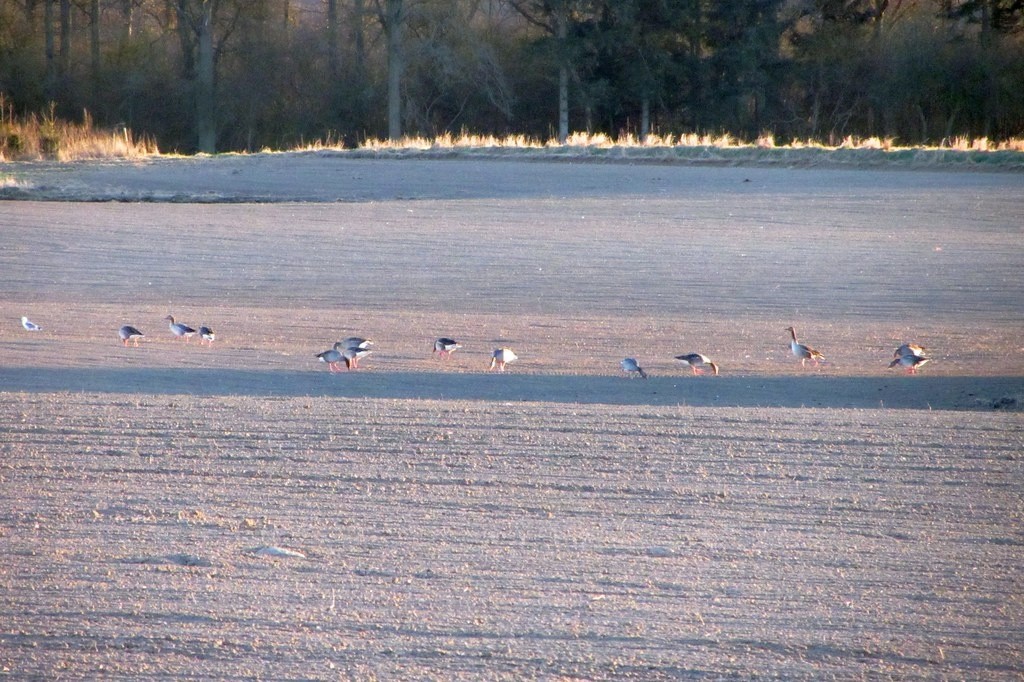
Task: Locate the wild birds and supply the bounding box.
[316,337,375,373]
[620,357,648,381]
[433,337,462,364]
[489,348,519,372]
[20,316,44,332]
[674,352,719,376]
[118,325,144,347]
[784,327,824,370]
[888,343,932,374]
[199,325,216,347]
[163,314,197,344]
